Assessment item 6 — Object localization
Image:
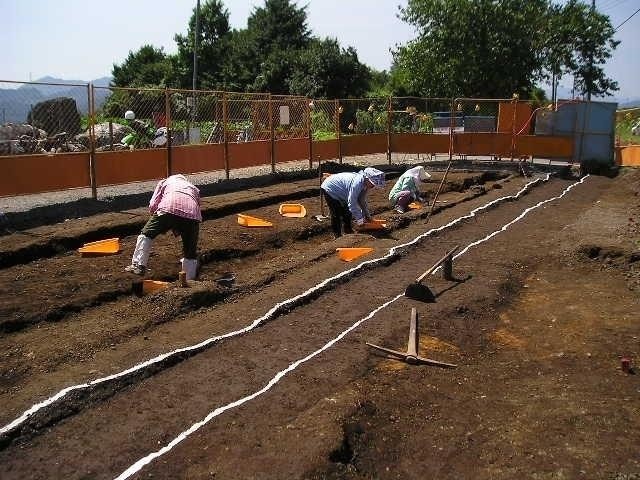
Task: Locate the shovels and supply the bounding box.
[405,244,461,303]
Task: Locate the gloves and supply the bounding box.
[357,218,363,226]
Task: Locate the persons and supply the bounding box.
[125,174,200,280]
[320,167,386,238]
[388,165,432,214]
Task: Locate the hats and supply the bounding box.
[365,168,385,188]
[418,166,431,180]
[124,110,135,119]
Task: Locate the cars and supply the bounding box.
[74,121,131,149]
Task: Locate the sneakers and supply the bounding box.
[394,205,404,213]
[125,264,145,276]
